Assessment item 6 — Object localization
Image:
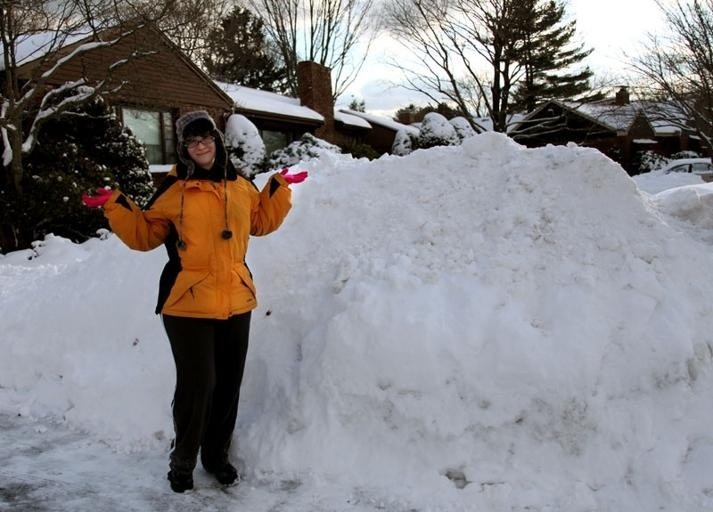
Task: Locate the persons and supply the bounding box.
[81,111,309,493]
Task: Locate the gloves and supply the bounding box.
[279,169,307,183]
[81,188,113,207]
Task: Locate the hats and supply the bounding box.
[176,111,228,179]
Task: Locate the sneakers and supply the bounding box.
[207,462,238,484]
[167,469,193,494]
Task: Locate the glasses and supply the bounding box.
[183,134,215,147]
